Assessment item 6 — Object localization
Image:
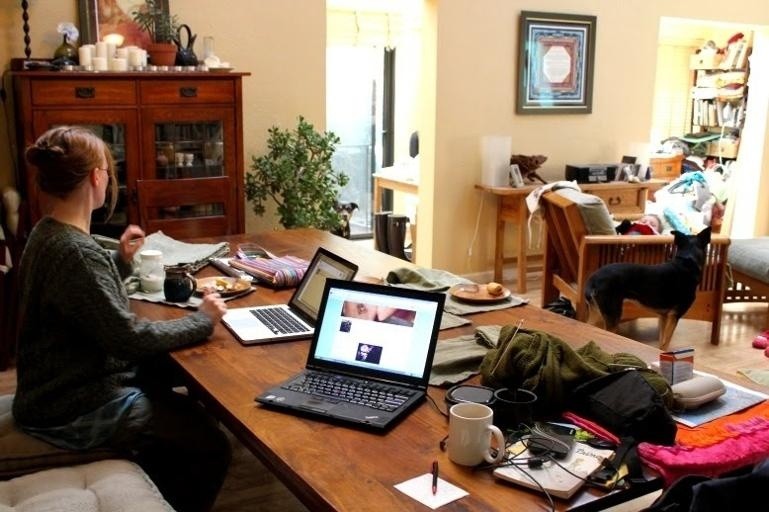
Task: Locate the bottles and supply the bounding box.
[450,283,511,303]
[138,249,162,295]
[53,30,79,65]
[203,36,216,58]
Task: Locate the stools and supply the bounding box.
[724,238,769,303]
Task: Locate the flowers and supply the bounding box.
[57,22,79,43]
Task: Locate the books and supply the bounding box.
[495,421,615,499]
[690,37,752,129]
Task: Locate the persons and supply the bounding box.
[11,124,231,512]
[617,215,661,234]
[342,301,416,324]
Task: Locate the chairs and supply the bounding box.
[0,394,175,512]
[539,188,732,351]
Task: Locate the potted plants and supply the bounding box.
[131,0,180,65]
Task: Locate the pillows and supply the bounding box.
[556,188,617,235]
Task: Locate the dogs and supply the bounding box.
[584,226,713,351]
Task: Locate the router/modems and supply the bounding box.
[526,421,576,459]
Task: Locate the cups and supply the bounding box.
[446,403,505,468]
[161,264,197,304]
[79,39,147,72]
[130,63,207,74]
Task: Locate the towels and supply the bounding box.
[122,275,141,294]
[386,268,450,292]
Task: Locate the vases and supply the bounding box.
[54,43,77,60]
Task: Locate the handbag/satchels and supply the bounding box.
[228,243,310,288]
[579,369,677,445]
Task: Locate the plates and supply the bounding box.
[210,65,234,73]
[193,274,251,295]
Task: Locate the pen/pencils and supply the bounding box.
[431,461,438,494]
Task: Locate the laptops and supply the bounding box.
[222,245,359,345]
[255,277,446,435]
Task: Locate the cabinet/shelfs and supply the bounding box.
[685,47,752,161]
[8,72,251,240]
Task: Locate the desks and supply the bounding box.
[473,180,668,293]
[110,227,769,511]
[370,174,419,265]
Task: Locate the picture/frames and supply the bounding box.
[515,10,597,115]
[78,0,172,64]
